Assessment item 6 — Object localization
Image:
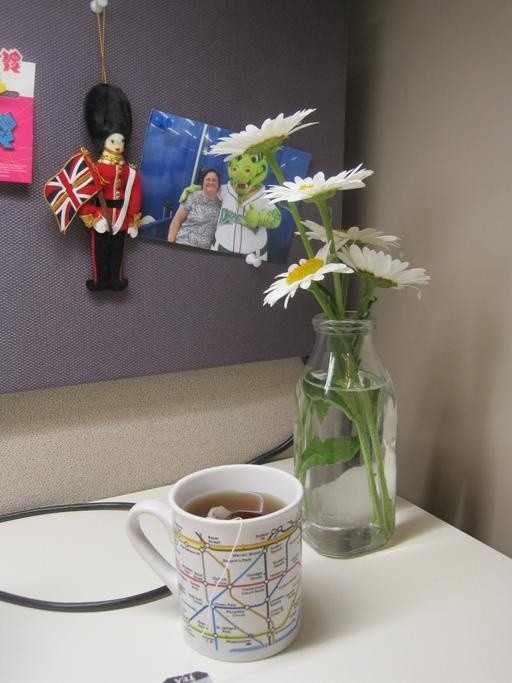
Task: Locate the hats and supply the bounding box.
[84,83,134,152]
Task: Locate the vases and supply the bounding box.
[292,315,397,559]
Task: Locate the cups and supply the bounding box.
[124,463,304,660]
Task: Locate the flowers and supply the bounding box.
[206,106,431,320]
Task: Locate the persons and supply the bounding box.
[168,169,222,250]
[76,83,142,293]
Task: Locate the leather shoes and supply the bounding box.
[108,279,129,291]
[86,279,106,291]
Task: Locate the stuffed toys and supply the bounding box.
[179,152,281,261]
